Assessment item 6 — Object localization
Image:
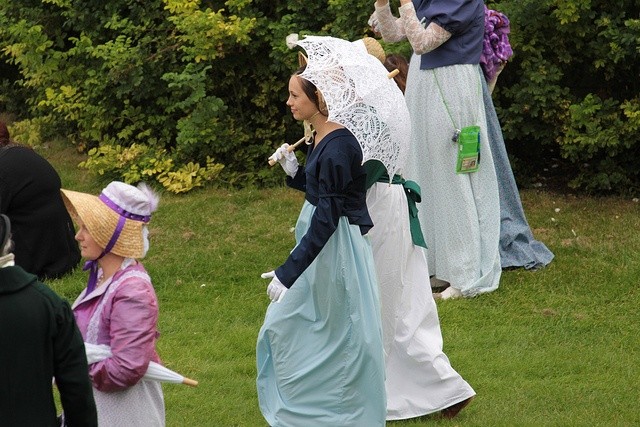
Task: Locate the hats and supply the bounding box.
[59,181,152,258]
[298,51,345,116]
[362,37,386,65]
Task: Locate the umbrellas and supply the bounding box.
[52,342,199,387]
[268,34,411,187]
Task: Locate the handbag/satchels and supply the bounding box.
[455,126,481,174]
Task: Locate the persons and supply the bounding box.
[0,214,98,427]
[51,181,165,427]
[256,63,387,427]
[384,53,409,96]
[374,0,501,300]
[361,37,476,421]
[480,0,555,269]
[1,120,81,281]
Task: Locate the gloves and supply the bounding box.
[260,269,288,303]
[267,143,299,179]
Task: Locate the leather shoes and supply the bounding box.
[439,286,461,299]
[437,397,474,419]
